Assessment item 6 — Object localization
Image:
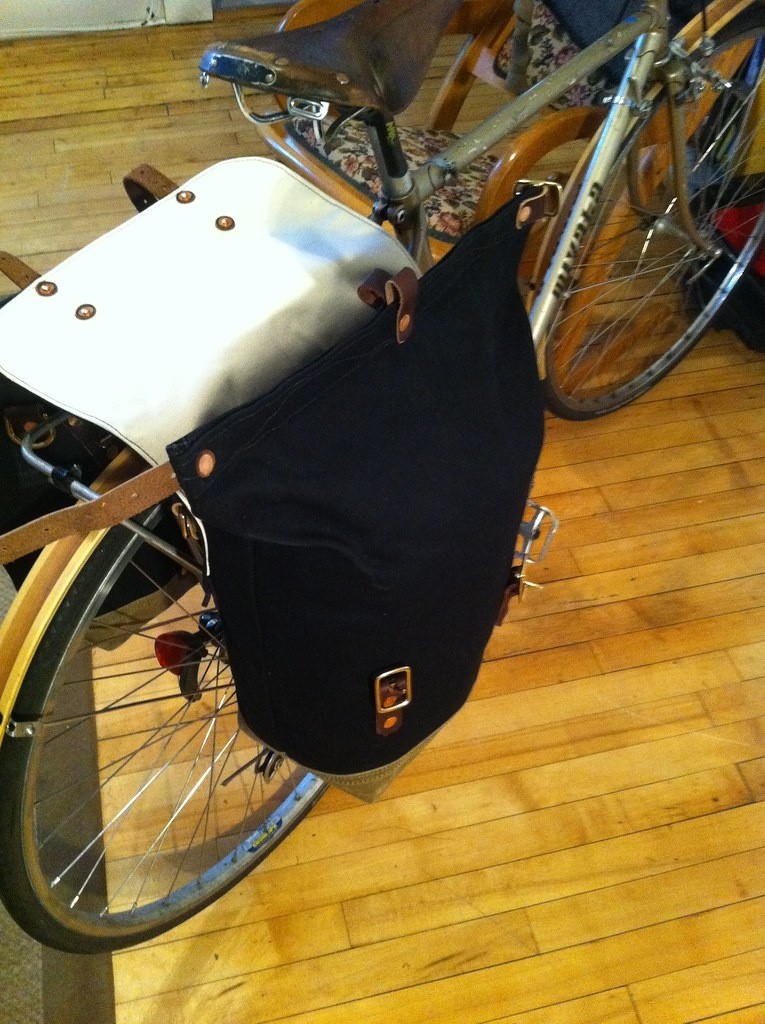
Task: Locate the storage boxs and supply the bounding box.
[689,170,765,356]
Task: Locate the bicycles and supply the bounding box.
[0,1,765,956]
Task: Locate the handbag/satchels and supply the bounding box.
[0,164,200,624]
[164,176,559,802]
